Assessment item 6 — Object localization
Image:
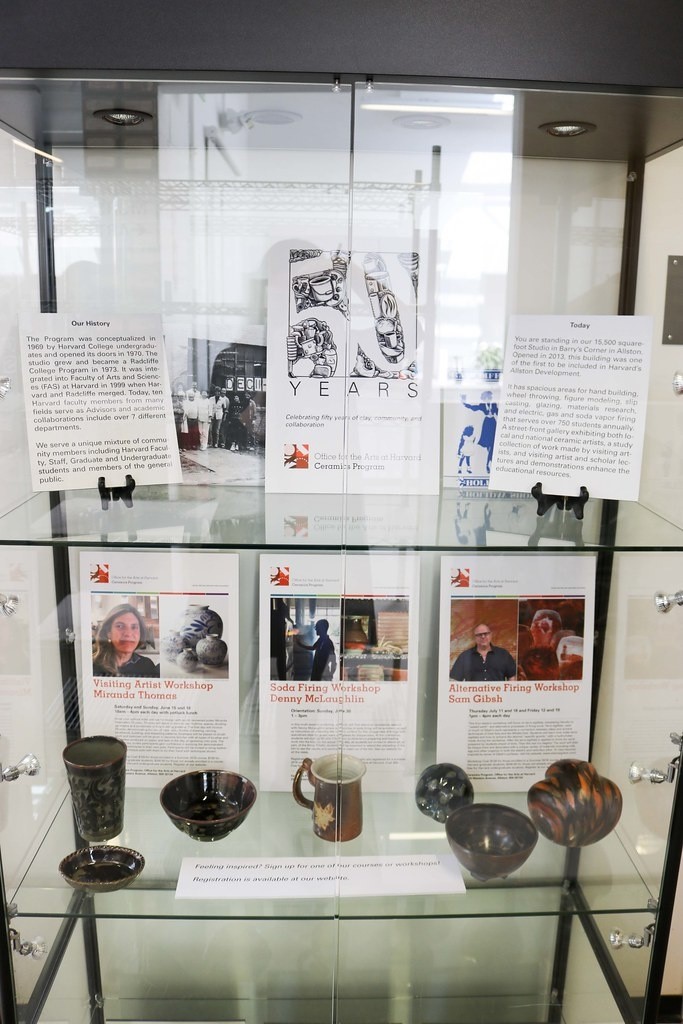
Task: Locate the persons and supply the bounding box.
[93,604,156,678]
[449,623,516,682]
[173,380,257,453]
[295,620,338,681]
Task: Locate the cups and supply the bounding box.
[293,752,366,842]
[63,736,127,842]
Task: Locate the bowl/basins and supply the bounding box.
[343,641,369,654]
[445,803,539,879]
[386,668,407,682]
[59,845,145,893]
[159,768,256,842]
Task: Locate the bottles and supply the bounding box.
[170,604,223,647]
[346,619,368,653]
[196,635,228,668]
[160,629,192,662]
[176,648,197,672]
